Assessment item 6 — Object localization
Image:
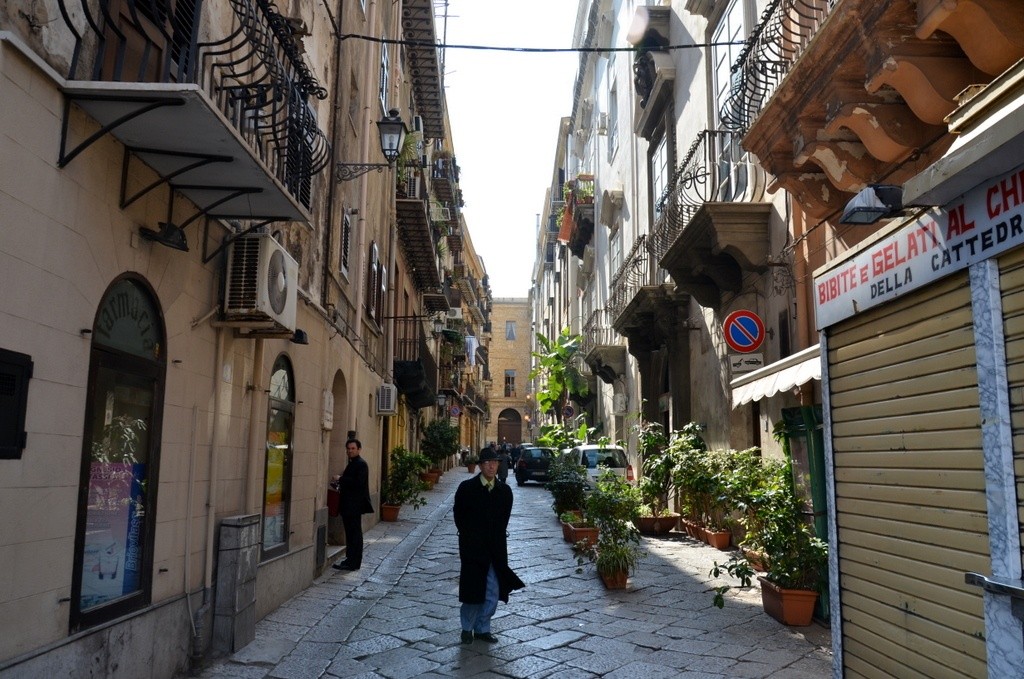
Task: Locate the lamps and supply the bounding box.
[340,109,408,182]
[840,183,905,226]
[435,390,446,406]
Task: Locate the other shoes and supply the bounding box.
[332,562,355,570]
[461,630,473,644]
[474,632,498,643]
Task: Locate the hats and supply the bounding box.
[475,447,503,464]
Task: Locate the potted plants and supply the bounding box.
[417,421,461,486]
[576,186,593,203]
[381,448,432,522]
[548,422,829,628]
[464,455,479,473]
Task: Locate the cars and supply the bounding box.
[566,444,635,503]
[506,443,573,482]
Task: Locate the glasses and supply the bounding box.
[482,461,499,466]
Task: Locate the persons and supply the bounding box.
[453,447,526,643]
[332,438,375,570]
[496,449,511,483]
[489,440,506,450]
[510,442,521,470]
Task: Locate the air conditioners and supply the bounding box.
[379,384,400,415]
[220,233,297,334]
[447,307,462,319]
[413,115,424,138]
